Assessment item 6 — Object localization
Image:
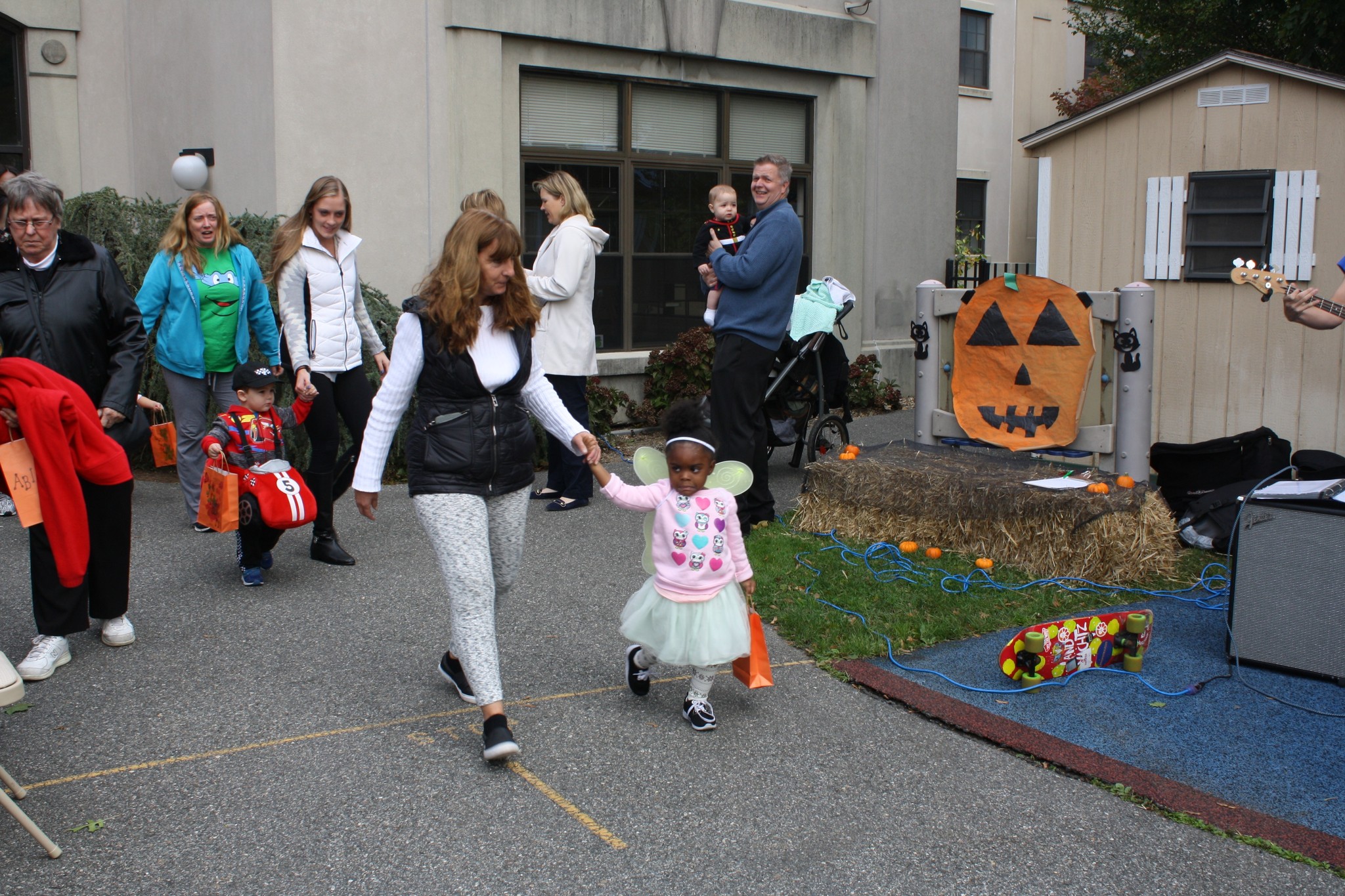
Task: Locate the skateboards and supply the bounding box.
[997,609,1154,693]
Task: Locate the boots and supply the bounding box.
[305,466,355,565]
[331,446,359,502]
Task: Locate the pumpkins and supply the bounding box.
[899,539,918,553]
[926,542,941,559]
[976,557,993,569]
[839,451,856,460]
[1087,482,1109,495]
[845,441,859,456]
[1116,472,1134,489]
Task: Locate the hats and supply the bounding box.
[233,362,282,393]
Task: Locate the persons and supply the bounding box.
[707,153,803,537]
[583,402,755,729]
[1283,255,1345,330]
[692,185,756,327]
[522,171,610,510]
[460,190,505,217]
[137,393,164,412]
[0,172,149,679]
[201,362,317,585]
[261,176,390,564]
[351,208,601,760]
[134,193,283,531]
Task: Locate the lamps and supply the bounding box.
[172,148,214,191]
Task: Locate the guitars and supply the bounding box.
[1229,257,1345,321]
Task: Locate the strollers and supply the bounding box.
[692,282,854,470]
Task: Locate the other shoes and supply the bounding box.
[438,651,478,704]
[481,715,520,760]
[530,489,563,500]
[194,521,213,532]
[545,496,590,513]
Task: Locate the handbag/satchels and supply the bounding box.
[196,450,240,533]
[1150,426,1292,518]
[733,592,774,690]
[149,405,177,468]
[0,423,45,530]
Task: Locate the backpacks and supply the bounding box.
[1180,475,1287,554]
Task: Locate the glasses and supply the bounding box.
[6,214,56,230]
[0,229,12,242]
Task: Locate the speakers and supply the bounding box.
[1226,496,1345,688]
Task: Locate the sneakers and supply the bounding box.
[0,491,17,516]
[95,613,135,646]
[683,692,717,732]
[625,645,651,696]
[241,568,265,586]
[261,551,272,570]
[16,633,72,680]
